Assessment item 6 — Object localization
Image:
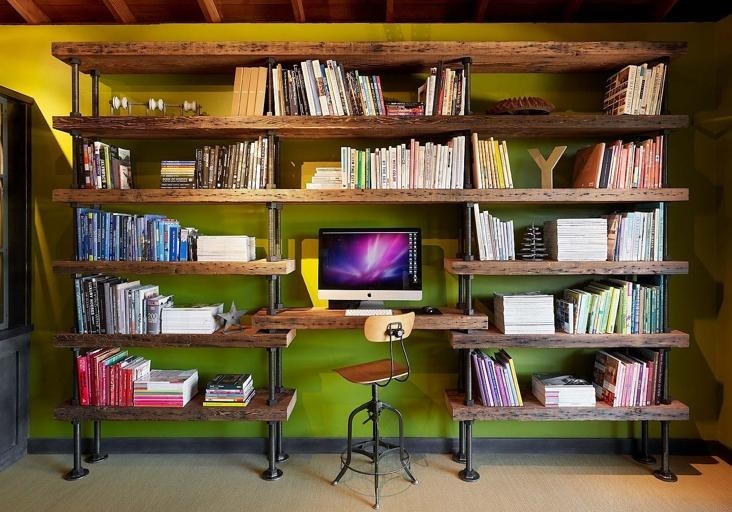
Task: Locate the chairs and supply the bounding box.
[333,310,416,510]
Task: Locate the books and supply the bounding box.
[272,59,468,191]
[468,202,669,263]
[495,277,670,335]
[468,133,514,190]
[573,136,670,189]
[231,66,271,117]
[469,349,670,410]
[161,135,271,188]
[71,207,197,262]
[203,375,256,406]
[70,275,224,335]
[604,62,669,116]
[72,347,199,411]
[72,134,131,189]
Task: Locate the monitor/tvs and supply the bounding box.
[318,227,423,309]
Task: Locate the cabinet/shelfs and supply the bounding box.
[51,39,692,484]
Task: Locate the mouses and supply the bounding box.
[422,305,434,313]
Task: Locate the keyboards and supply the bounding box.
[344,309,392,317]
